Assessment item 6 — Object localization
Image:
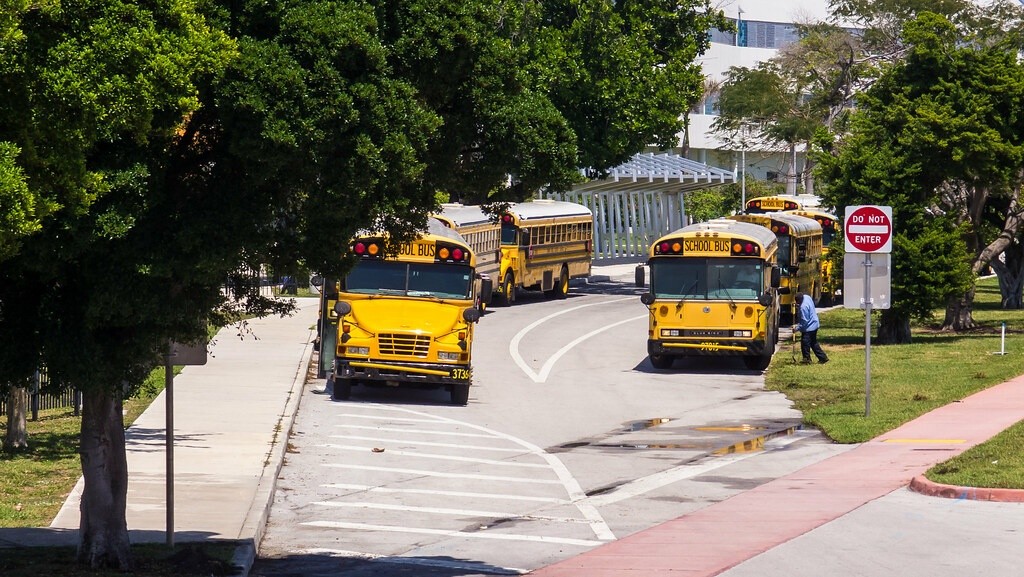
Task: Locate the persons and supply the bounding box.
[792,292,829,365]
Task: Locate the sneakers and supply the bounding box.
[816,357,829,364]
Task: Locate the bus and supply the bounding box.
[743,193,837,220]
[722,212,823,318]
[492,197,594,308]
[315,215,481,408]
[782,210,841,302]
[635,219,781,370]
[425,203,501,314]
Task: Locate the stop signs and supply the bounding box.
[847,208,892,252]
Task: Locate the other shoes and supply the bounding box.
[799,358,812,365]
